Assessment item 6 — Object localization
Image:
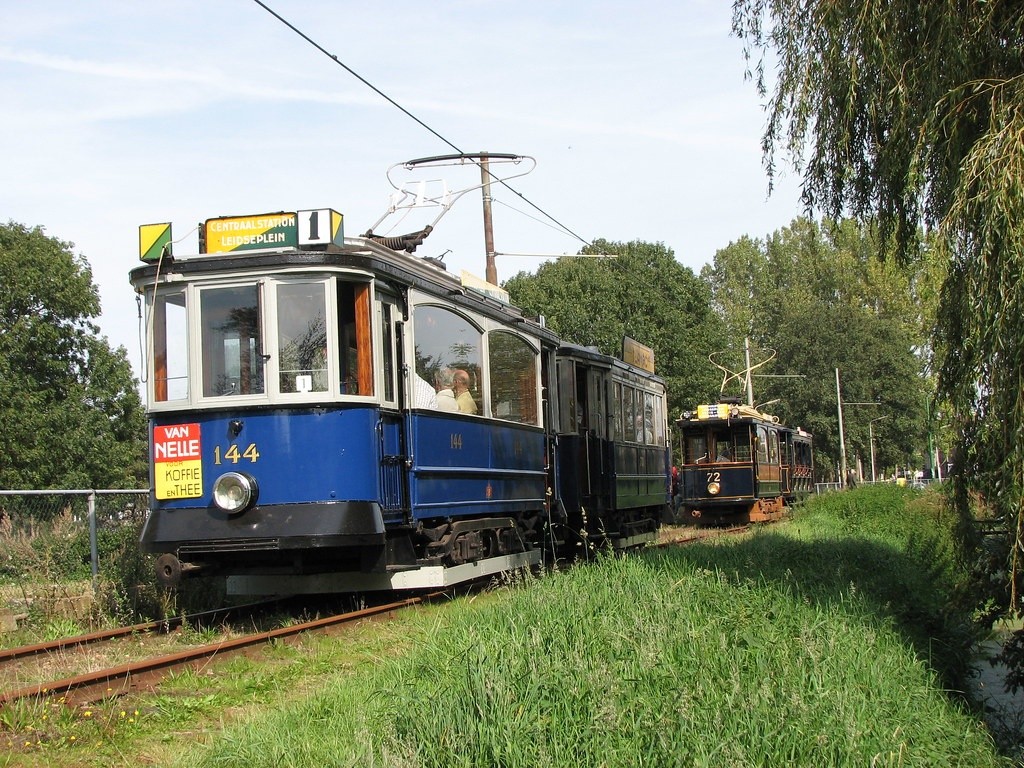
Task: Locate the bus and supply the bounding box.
[128,209,675,611]
[682,397,818,526]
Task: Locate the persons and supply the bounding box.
[634,415,653,444]
[572,396,584,424]
[414,365,480,415]
